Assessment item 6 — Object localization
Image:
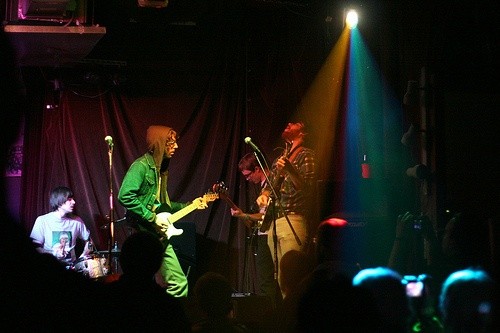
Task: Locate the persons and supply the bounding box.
[29,186,94,269]
[117,126,206,298]
[103,232,192,333]
[190,120,500,333]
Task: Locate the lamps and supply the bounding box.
[138,0,168,8]
[401,123,426,145]
[403,80,425,107]
[406,163,426,182]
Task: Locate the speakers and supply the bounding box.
[3,0,94,27]
[170,222,197,296]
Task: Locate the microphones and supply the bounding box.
[102,216,126,231]
[105,136,114,147]
[245,137,260,153]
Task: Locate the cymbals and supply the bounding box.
[92,249,121,253]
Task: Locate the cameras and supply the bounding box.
[403,276,425,299]
[409,216,424,233]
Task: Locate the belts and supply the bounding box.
[276,210,296,218]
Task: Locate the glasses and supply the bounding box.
[243,172,253,178]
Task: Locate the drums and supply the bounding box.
[73,257,108,278]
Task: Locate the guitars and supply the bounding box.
[260,140,294,233]
[211,181,259,229]
[137,180,230,253]
